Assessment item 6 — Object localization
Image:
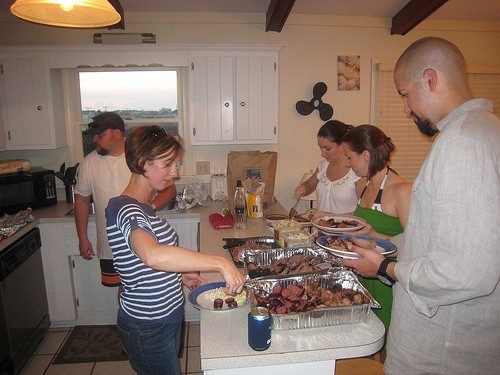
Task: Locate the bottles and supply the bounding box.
[233,180,247,230]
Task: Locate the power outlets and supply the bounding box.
[196,161,210,175]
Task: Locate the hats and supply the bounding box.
[83,113,125,134]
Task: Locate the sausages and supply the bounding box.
[214,298,238,308]
[318,218,348,228]
[263,284,309,313]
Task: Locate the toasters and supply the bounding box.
[210,173,229,201]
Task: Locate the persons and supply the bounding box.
[343,35,500,375]
[294,120,361,217]
[73,112,177,286]
[343,125,412,363]
[105,126,245,375]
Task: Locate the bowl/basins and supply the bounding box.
[266,221,315,249]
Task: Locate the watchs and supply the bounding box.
[376,259,397,286]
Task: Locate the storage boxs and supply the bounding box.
[273,219,313,248]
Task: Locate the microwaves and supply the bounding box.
[0,166,57,216]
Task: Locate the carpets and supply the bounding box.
[52,322,186,365]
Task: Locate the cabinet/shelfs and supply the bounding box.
[0,46,65,151]
[37,221,200,328]
[186,45,284,146]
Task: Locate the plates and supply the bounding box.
[266,214,289,223]
[310,215,367,234]
[188,281,253,312]
[292,213,312,225]
[316,234,397,260]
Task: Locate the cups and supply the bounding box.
[66,185,76,204]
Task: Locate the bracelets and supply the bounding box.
[150,204,155,210]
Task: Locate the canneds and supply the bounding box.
[250,306,270,321]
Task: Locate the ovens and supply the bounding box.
[0,227,52,375]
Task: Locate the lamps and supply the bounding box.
[10,1,121,28]
[94,33,157,44]
